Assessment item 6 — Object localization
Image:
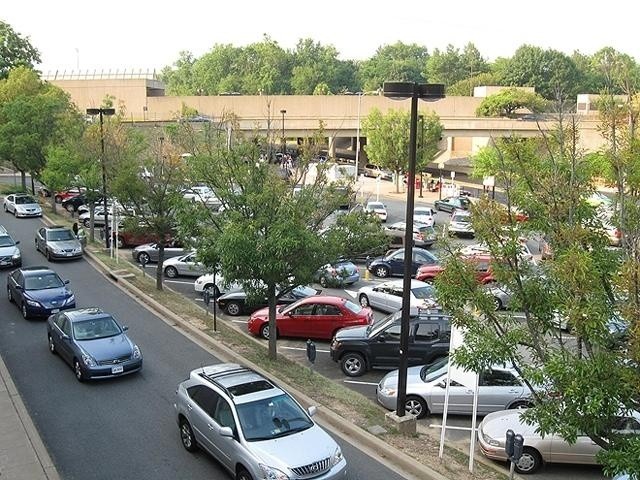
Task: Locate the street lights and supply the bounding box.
[85,107,122,249]
[280,110,287,151]
[196,89,204,97]
[257,87,264,96]
[227,123,232,154]
[353,90,381,180]
[383,78,449,416]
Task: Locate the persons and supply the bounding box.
[73,223,78,235]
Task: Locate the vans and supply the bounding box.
[361,162,393,180]
[0,224,23,269]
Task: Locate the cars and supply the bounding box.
[6,265,77,322]
[248,296,375,340]
[215,285,324,316]
[44,305,144,385]
[589,216,620,246]
[2,193,42,219]
[360,280,450,317]
[34,224,86,264]
[604,295,632,346]
[377,354,561,421]
[477,398,640,475]
[551,297,580,333]
[484,276,548,311]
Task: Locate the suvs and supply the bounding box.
[329,306,453,379]
[173,361,349,480]
